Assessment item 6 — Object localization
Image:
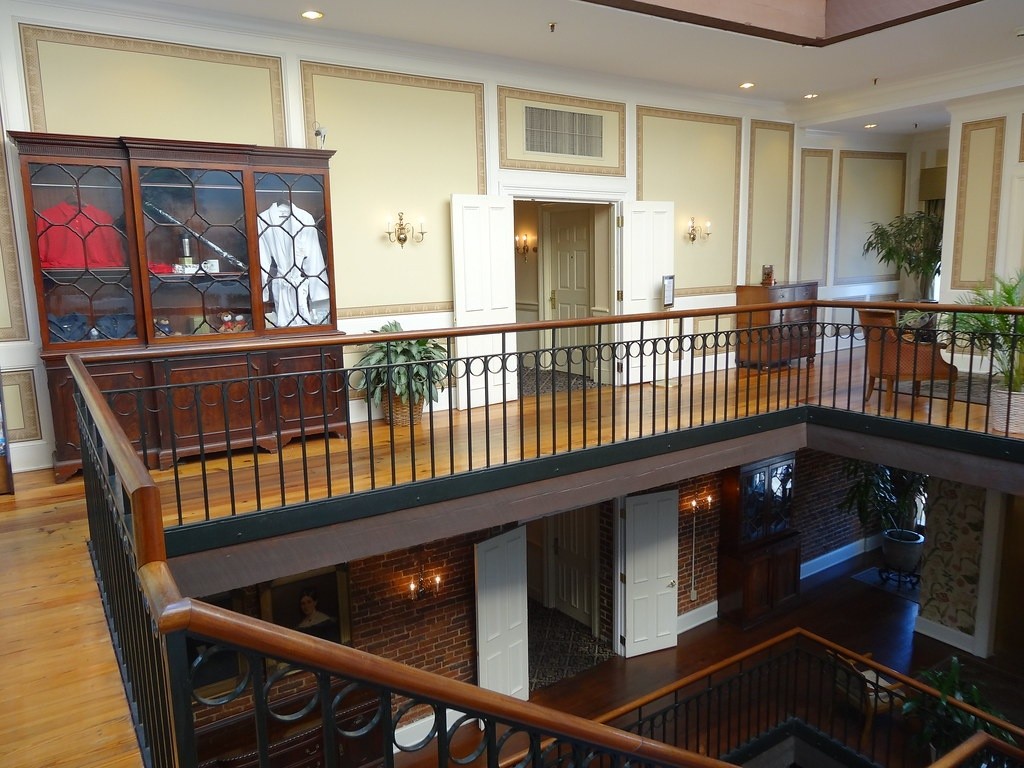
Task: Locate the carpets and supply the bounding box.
[850,565,920,602]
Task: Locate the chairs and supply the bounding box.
[856,308,958,413]
[826,649,912,753]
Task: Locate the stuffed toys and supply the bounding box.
[153,314,182,337]
[216,311,249,332]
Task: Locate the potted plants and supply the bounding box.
[901,653,1020,768]
[862,210,943,329]
[836,459,927,575]
[352,318,447,427]
[897,268,1024,433]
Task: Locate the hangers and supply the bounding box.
[277,185,291,218]
[63,185,88,207]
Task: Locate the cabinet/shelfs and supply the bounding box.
[5,128,352,484]
[194,679,384,768]
[716,452,802,635]
[735,280,818,372]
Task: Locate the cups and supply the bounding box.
[761,264,775,285]
[202,260,220,273]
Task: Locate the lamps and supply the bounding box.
[685,215,712,245]
[384,211,428,249]
[690,494,713,518]
[408,558,443,601]
[516,233,529,262]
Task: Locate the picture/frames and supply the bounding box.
[256,563,353,683]
[187,586,254,706]
